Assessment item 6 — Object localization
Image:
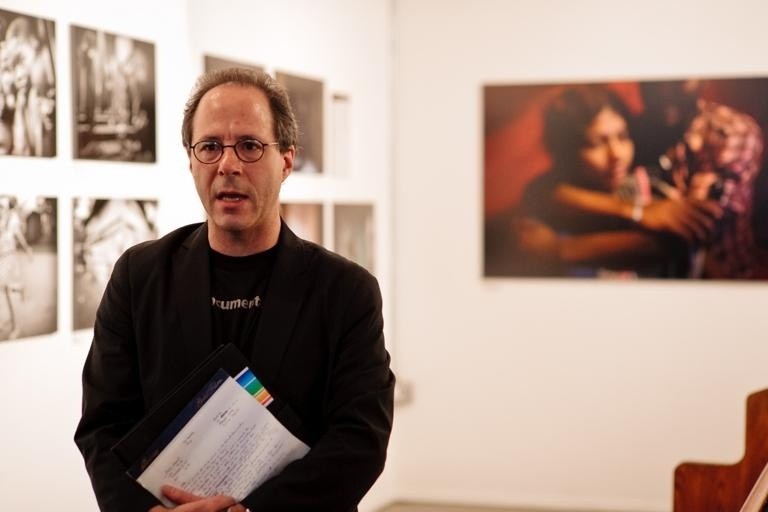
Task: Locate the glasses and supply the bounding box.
[188,140,280,163]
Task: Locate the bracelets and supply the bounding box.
[628,205,642,225]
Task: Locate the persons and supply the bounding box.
[0,195,32,338]
[78,70,392,512]
[526,79,759,276]
[0,66,47,155]
[510,87,715,276]
